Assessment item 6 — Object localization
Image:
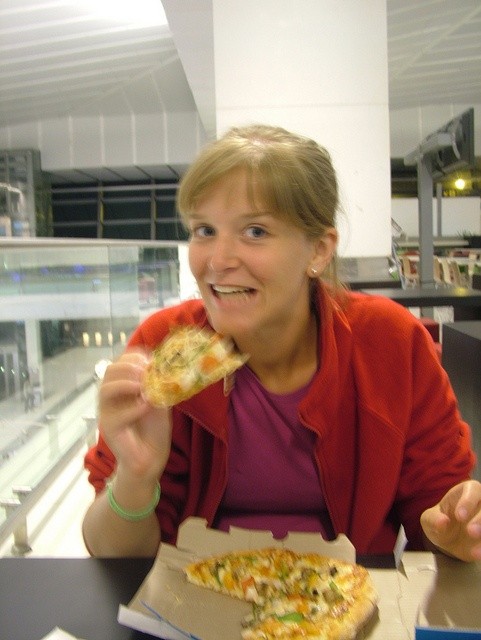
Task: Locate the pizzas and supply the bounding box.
[139,323,251,411]
[185,544,380,640]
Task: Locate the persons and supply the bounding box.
[82,124,481,563]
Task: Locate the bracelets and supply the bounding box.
[108,478,161,521]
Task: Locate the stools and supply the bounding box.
[434,256,443,288]
[468,254,477,286]
[451,260,468,287]
[441,257,452,287]
[403,257,418,288]
